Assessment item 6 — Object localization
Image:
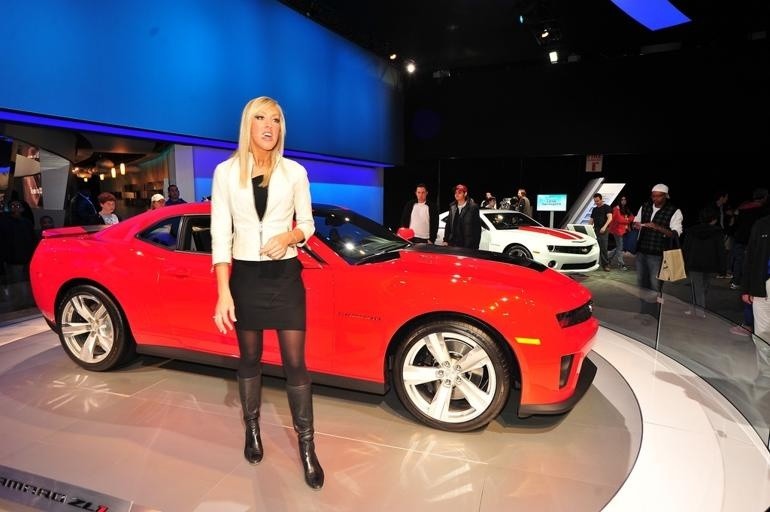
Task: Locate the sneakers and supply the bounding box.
[728,325,752,336]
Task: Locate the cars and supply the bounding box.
[27,198,607,437]
[433,206,604,280]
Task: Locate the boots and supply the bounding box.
[284,375,324,490]
[237,367,264,464]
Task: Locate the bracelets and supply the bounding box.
[289,229,298,244]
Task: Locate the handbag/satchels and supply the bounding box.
[656,248,687,282]
[623,230,638,255]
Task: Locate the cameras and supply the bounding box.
[640,224,646,227]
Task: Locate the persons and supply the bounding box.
[150,193,166,209]
[2,199,33,280]
[67,180,97,226]
[208,97,324,490]
[39,216,56,230]
[479,189,531,226]
[96,192,121,226]
[400,183,439,245]
[587,183,770,387]
[166,184,187,206]
[442,184,481,250]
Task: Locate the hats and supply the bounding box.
[651,183,671,200]
[151,193,166,202]
